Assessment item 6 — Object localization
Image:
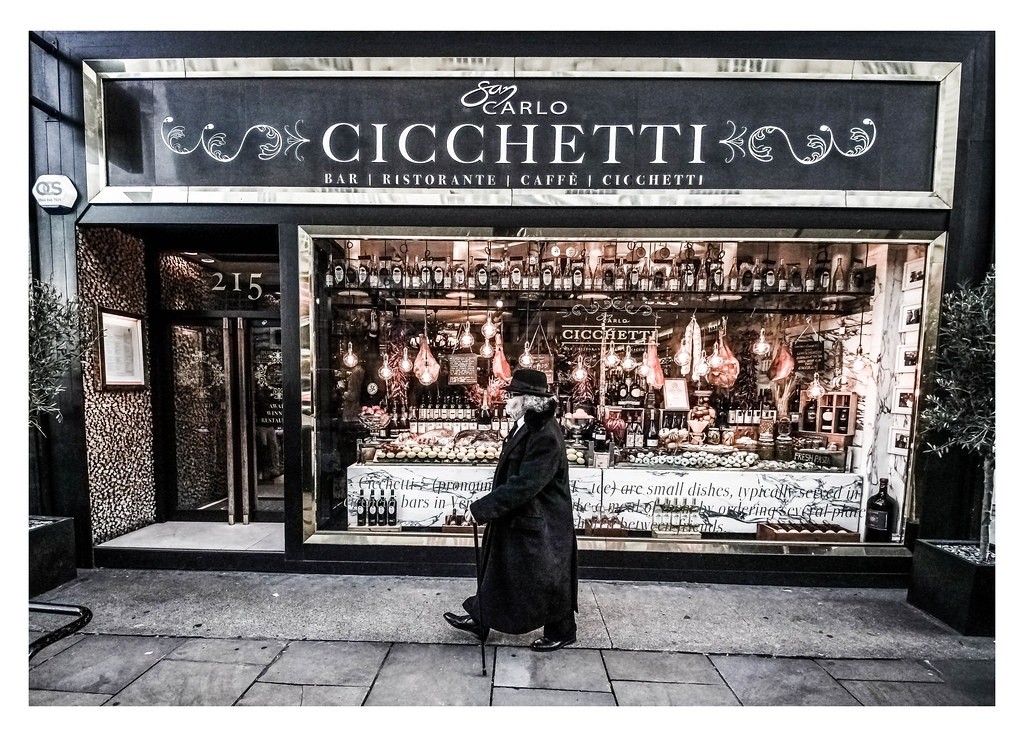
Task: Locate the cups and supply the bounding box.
[777,421,793,436]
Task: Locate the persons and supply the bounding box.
[443,368,579,653]
[254,376,280,478]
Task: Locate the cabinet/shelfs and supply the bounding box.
[799,389,858,448]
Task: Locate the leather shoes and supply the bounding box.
[443,612,490,641]
[531,636,576,652]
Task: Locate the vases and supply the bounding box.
[603,410,626,447]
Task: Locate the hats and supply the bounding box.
[500,368,554,397]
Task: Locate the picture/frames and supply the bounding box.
[885,257,926,457]
[92,304,149,393]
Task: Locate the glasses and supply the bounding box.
[507,391,525,398]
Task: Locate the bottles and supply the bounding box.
[321,251,858,291]
[492,406,515,438]
[387,488,398,526]
[605,371,649,408]
[837,399,850,433]
[645,409,660,451]
[652,495,702,532]
[377,489,387,527]
[477,388,493,433]
[710,380,777,427]
[356,489,367,526]
[663,356,672,378]
[368,489,378,527]
[708,428,735,446]
[379,389,476,438]
[588,410,644,453]
[766,514,850,533]
[821,395,834,436]
[802,398,818,432]
[663,411,688,429]
[792,379,801,413]
[761,351,771,372]
[864,477,900,543]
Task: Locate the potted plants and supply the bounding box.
[907,263,995,637]
[28,276,107,597]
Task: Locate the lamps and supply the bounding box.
[341,238,870,399]
